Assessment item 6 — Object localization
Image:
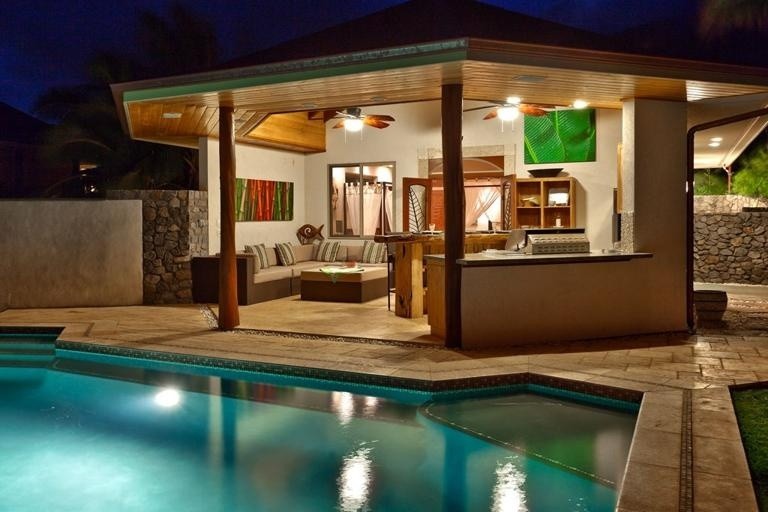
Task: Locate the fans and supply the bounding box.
[310,107,396,132]
[463,98,573,121]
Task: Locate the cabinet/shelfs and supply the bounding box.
[512,173,575,230]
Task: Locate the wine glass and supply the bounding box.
[428,223,435,235]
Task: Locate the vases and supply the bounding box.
[695,289,727,322]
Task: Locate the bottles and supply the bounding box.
[554,213,562,226]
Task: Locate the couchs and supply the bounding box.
[190,241,389,306]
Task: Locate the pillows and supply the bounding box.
[362,239,387,264]
[245,242,298,269]
[317,240,341,262]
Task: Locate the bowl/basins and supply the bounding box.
[527,168,562,177]
